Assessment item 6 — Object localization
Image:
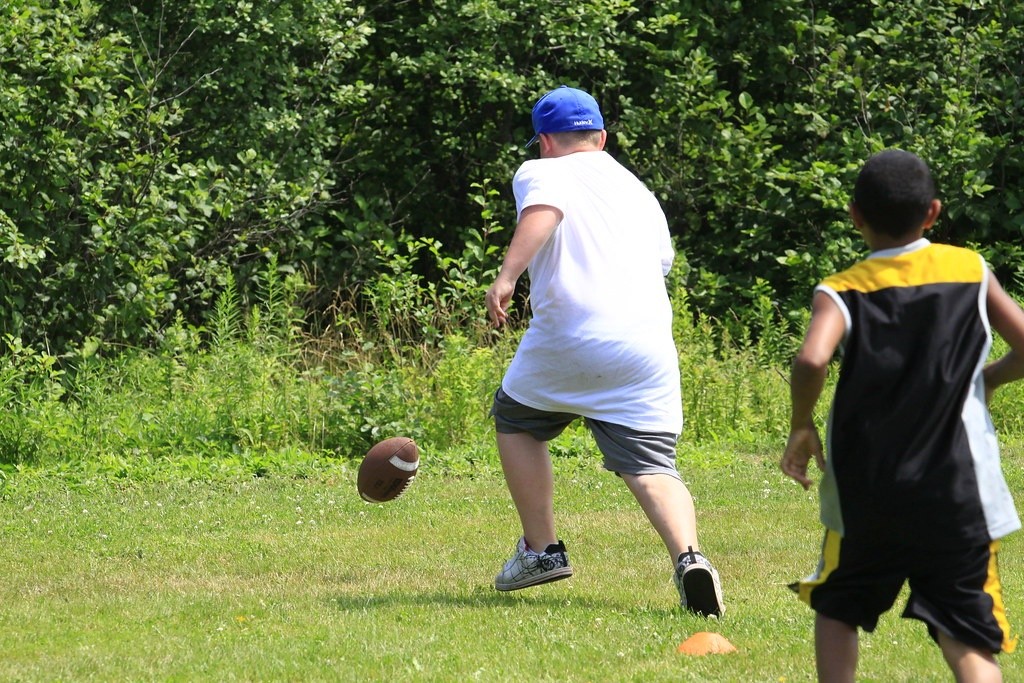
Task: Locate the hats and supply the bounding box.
[524,84,605,147]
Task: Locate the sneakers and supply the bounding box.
[673,546,726,615]
[495,537,574,592]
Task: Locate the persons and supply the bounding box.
[782,144,1024,683]
[487,83,724,615]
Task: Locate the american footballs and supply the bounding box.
[357,437,419,503]
